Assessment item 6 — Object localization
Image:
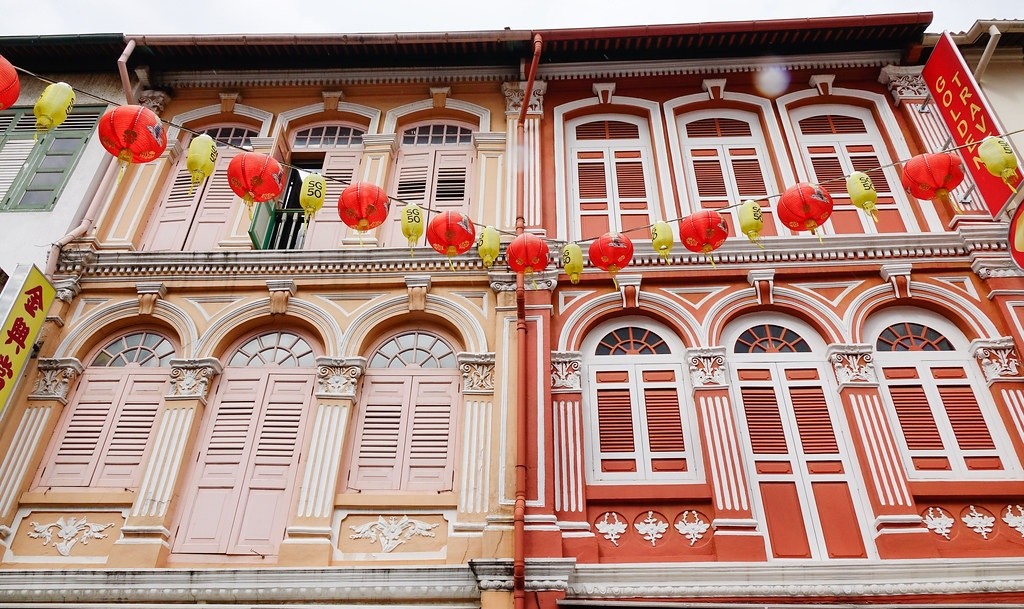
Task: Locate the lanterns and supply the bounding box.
[0,55,1018,284]
